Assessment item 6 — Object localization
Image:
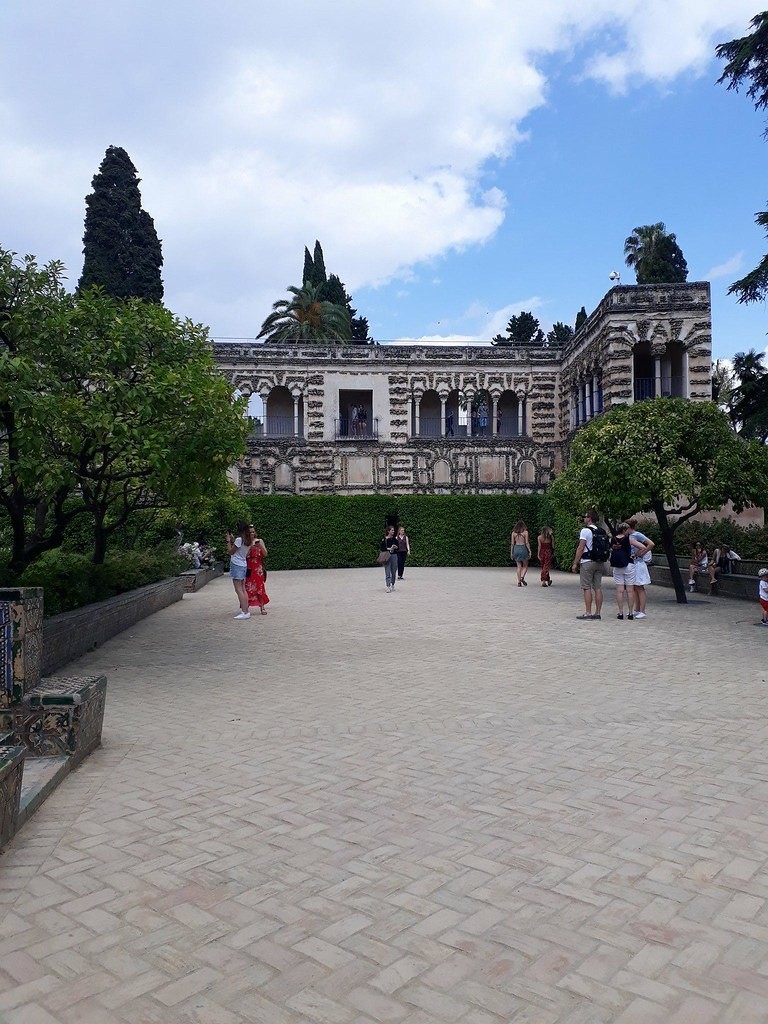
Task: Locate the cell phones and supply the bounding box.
[227,528,231,536]
[255,540,260,544]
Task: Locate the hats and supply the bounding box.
[758,568,768,576]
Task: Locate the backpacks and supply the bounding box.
[611,536,629,567]
[583,527,610,562]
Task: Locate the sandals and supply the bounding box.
[261,608,267,615]
[518,583,521,587]
[520,577,527,586]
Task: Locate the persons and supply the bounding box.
[688,541,708,593]
[226,519,268,620]
[537,526,554,587]
[510,520,532,587]
[610,519,655,620]
[396,527,410,580]
[758,568,768,625]
[572,510,604,619]
[194,541,217,572]
[708,544,741,595]
[380,526,399,593]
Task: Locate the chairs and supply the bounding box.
[0,586,108,850]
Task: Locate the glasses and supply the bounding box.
[723,548,727,550]
[583,516,590,518]
[249,524,254,527]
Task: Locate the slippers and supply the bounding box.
[707,592,713,596]
[710,579,717,585]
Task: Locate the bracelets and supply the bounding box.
[227,540,231,544]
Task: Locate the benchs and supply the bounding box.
[604,550,768,602]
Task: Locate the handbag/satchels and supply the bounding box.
[246,568,251,578]
[642,549,652,562]
[376,539,391,564]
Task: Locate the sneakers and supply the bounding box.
[689,580,695,585]
[690,584,696,592]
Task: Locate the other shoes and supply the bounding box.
[392,585,395,592]
[761,619,768,626]
[576,614,593,621]
[233,612,251,619]
[616,613,623,619]
[398,576,403,579]
[628,614,633,619]
[626,610,639,616]
[549,580,552,586]
[635,612,647,619]
[593,614,601,621]
[542,583,548,587]
[386,587,391,593]
